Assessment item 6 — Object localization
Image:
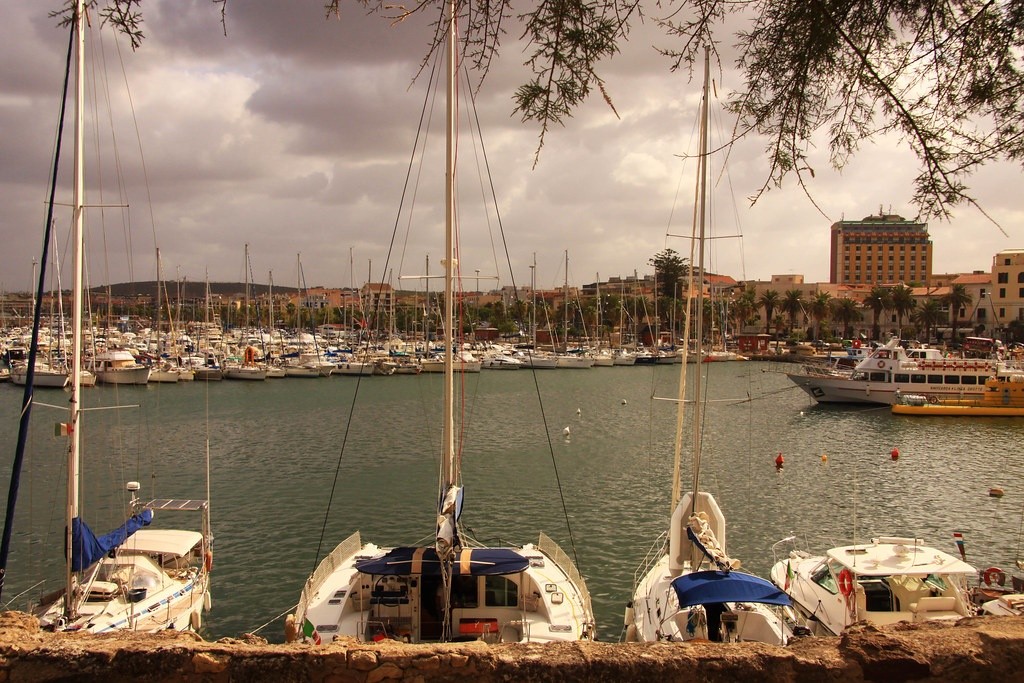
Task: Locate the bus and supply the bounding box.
[962,336,994,353]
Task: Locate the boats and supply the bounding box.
[770,532,1024,633]
[890,376,1024,416]
[784,328,1024,408]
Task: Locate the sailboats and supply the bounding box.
[0,232,814,389]
[0,0,213,636]
[282,1,599,647]
[619,38,814,648]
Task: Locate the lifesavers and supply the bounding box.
[207,551,213,573]
[878,360,886,368]
[984,568,1006,587]
[975,350,982,357]
[929,396,938,404]
[838,569,853,596]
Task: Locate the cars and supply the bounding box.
[811,339,830,347]
